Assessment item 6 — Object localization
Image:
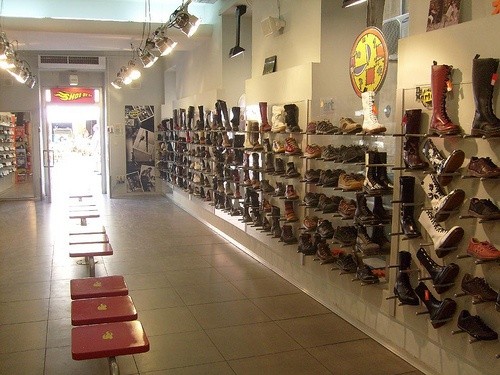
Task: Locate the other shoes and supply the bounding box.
[495,295,500,311]
[0,120,32,176]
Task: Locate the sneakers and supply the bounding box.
[457,310,498,340]
[469,157,500,177]
[466,238,500,260]
[468,198,500,219]
[461,274,498,301]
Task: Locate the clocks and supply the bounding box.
[349,26,389,98]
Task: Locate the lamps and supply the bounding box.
[139,48,159,69]
[153,28,178,57]
[341,0,366,8]
[228,5,246,58]
[0,34,36,88]
[111,63,141,90]
[167,6,200,38]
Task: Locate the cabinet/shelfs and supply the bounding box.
[0,112,33,193]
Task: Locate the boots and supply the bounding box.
[156,91,394,284]
[394,251,419,305]
[400,176,421,236]
[471,53,500,136]
[403,109,429,169]
[418,210,465,258]
[421,139,465,187]
[428,60,461,134]
[420,174,465,222]
[417,248,459,294]
[415,282,457,328]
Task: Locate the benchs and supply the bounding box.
[68,203,99,226]
[69,196,91,202]
[70,224,113,277]
[71,275,149,375]
